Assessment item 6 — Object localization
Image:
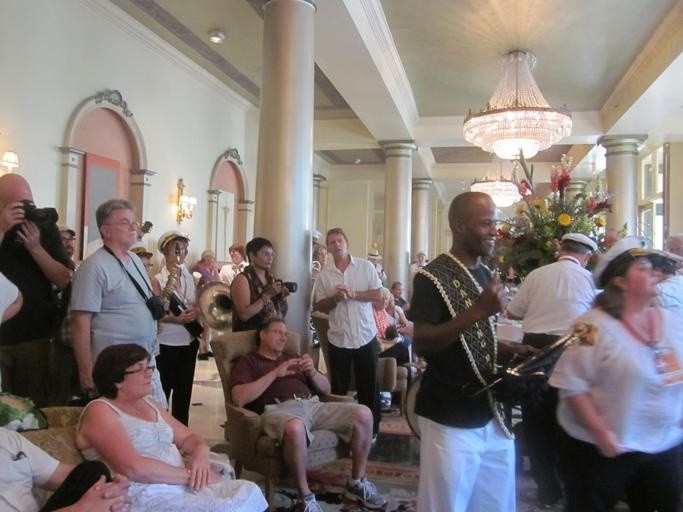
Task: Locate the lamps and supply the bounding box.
[471,155,532,208]
[177,178,196,223]
[0,151,18,173]
[463,53,573,160]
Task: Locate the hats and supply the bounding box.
[158,231,191,250]
[58,226,75,236]
[562,233,598,252]
[128,242,153,258]
[593,238,659,288]
[368,249,382,259]
[645,248,683,274]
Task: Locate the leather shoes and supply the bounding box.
[199,354,208,360]
[208,352,215,356]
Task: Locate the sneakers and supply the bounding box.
[345,475,388,508]
[296,493,325,512]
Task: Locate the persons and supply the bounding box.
[642,249,682,310]
[1,428,131,512]
[0,275,21,333]
[317,227,382,450]
[389,294,419,362]
[77,343,270,511]
[219,245,247,285]
[230,318,387,512]
[390,283,407,316]
[56,227,88,402]
[74,199,168,425]
[151,231,203,422]
[410,251,428,296]
[365,246,385,282]
[507,233,598,503]
[547,250,682,512]
[371,289,412,391]
[193,250,221,360]
[231,238,290,333]
[0,174,71,404]
[408,190,537,512]
[312,246,327,370]
[130,248,152,279]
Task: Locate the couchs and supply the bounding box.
[310,312,408,417]
[210,329,356,509]
[0,406,235,512]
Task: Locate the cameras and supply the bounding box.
[147,296,165,320]
[20,200,57,229]
[274,279,297,298]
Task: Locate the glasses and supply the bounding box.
[127,365,156,374]
[101,221,137,225]
[61,236,76,243]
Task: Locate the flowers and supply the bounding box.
[495,150,631,289]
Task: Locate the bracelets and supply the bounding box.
[352,288,356,299]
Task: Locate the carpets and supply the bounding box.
[177,404,628,512]
